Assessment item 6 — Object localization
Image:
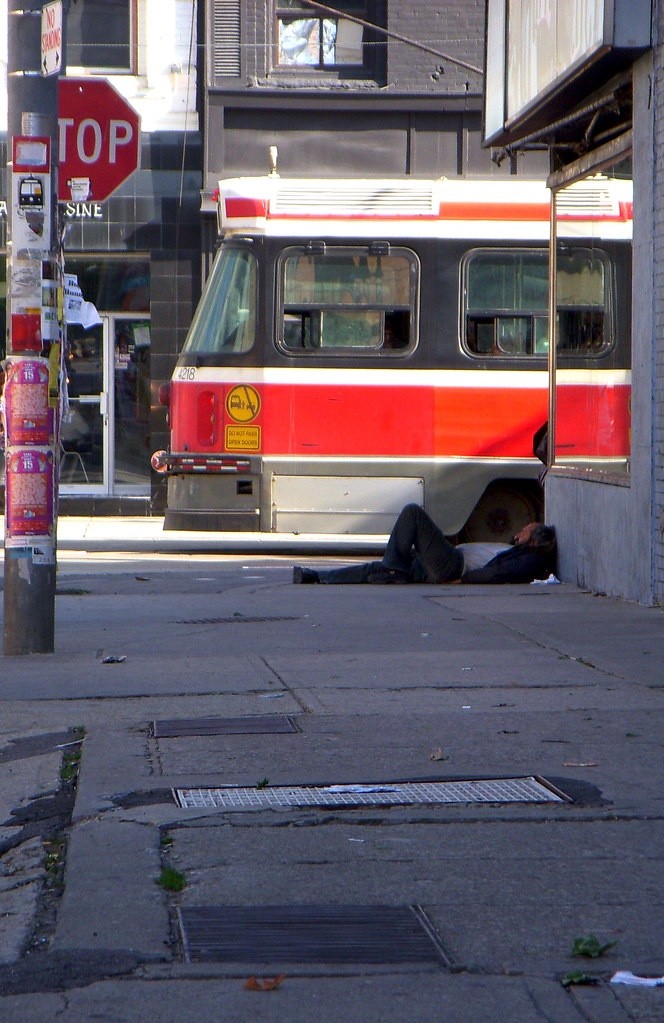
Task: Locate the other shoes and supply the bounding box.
[293,566,318,584]
[367,569,406,583]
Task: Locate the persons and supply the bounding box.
[293,503,558,584]
[60,400,126,467]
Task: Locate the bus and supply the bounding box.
[152,142,637,554]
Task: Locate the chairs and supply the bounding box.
[58,405,101,483]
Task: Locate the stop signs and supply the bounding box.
[53,74,140,205]
